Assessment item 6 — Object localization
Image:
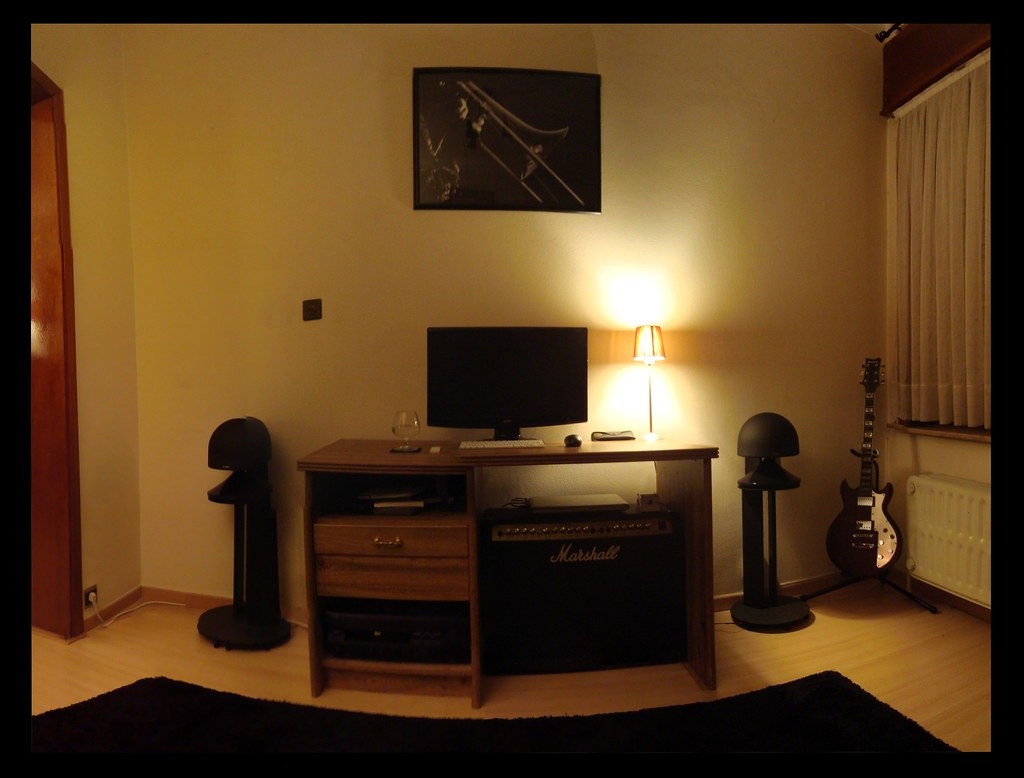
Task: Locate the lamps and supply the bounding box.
[632,326,665,440]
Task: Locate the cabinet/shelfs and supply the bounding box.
[296,433,720,709]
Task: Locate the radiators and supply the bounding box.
[904,472,990,615]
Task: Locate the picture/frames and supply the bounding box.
[412,67,602,213]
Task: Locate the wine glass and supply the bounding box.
[390,410,421,451]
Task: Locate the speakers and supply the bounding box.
[480,501,689,676]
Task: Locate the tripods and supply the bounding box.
[800,449,937,613]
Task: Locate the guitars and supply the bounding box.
[825,354,904,578]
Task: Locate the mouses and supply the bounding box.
[564,435,581,447]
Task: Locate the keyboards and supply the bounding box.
[459,440,546,449]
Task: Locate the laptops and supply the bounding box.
[526,495,630,514]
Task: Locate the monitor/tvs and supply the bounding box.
[427,326,587,440]
